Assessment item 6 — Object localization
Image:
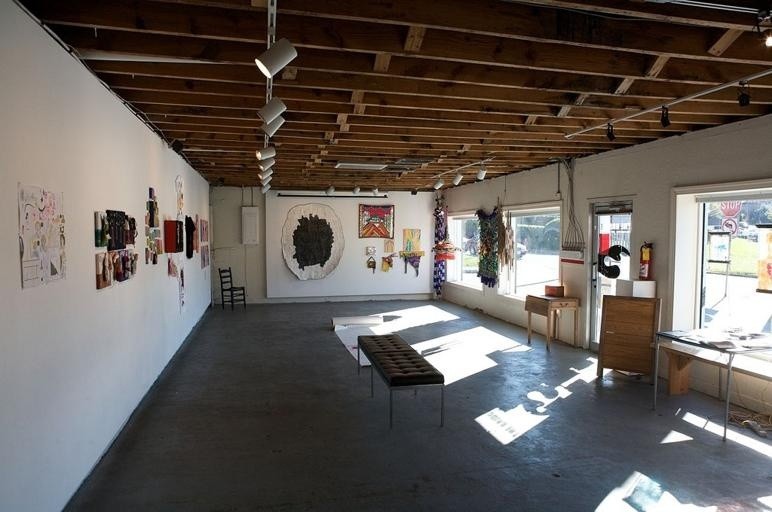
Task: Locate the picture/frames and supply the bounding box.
[200,220,208,242]
[359,204,394,238]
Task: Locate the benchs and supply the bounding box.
[357,333,445,427]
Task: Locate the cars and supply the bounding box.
[465,233,527,259]
[736,222,758,242]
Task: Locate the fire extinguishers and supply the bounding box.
[639,240,654,281]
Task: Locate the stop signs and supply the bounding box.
[720,200,741,217]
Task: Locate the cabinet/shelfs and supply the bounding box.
[615,279,657,377]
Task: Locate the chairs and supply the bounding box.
[218,267,246,310]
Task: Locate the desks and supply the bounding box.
[650,330,771,441]
[524,295,580,352]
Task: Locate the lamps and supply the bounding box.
[563,0,772,141]
[320,184,379,194]
[254,0,298,194]
[430,155,496,189]
